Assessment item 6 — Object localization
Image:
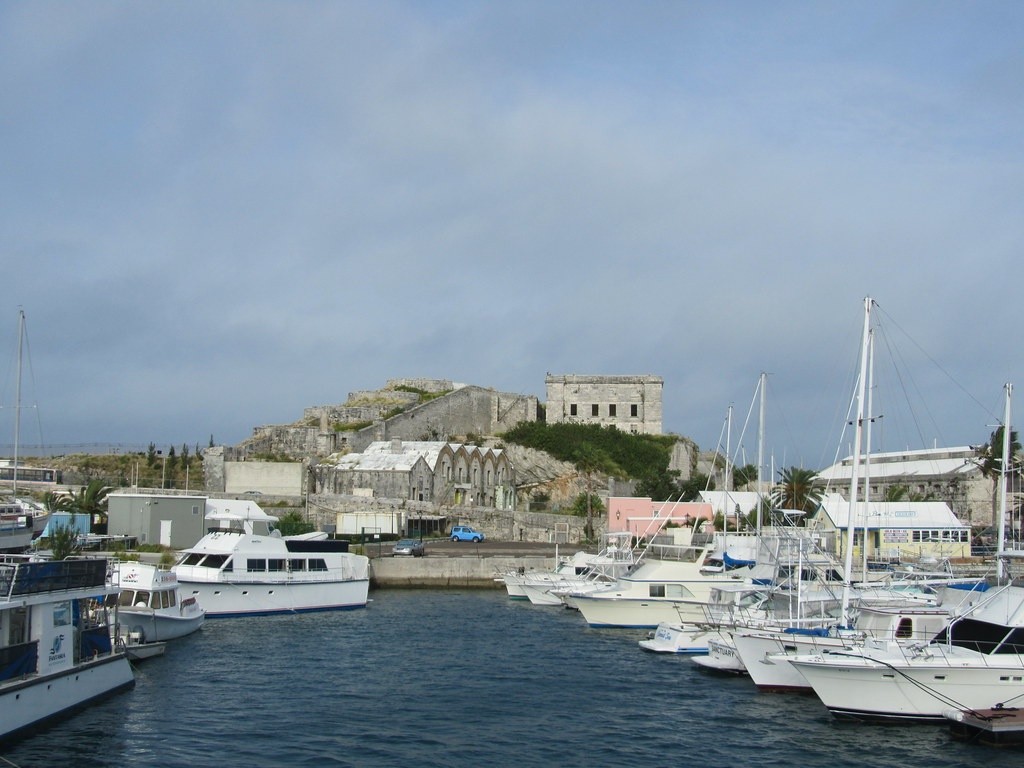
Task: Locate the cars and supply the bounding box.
[393,538,424,557]
[451,526,485,543]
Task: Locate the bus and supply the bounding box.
[0,466,62,487]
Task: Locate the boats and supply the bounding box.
[172,527,370,616]
[107,624,166,659]
[0,558,133,734]
[88,562,205,641]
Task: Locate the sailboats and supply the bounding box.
[493,294,1024,718]
[0,307,52,554]
